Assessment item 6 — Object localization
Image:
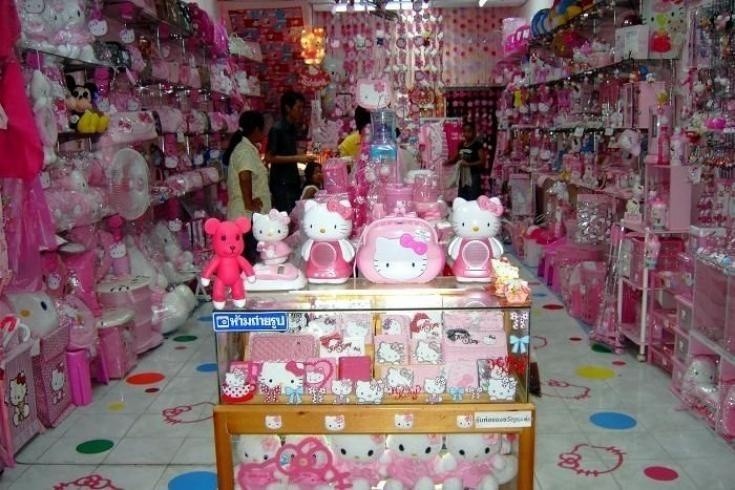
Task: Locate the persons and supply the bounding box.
[300,162,321,196]
[222,111,274,267]
[338,106,372,166]
[394,129,417,183]
[443,123,486,201]
[266,91,317,215]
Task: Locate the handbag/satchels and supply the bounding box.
[353,216,442,284]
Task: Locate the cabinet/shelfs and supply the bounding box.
[314,86,505,231]
[491,4,735,451]
[2,2,270,467]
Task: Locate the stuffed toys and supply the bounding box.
[196,220,255,311]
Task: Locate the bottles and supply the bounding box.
[372,110,396,145]
